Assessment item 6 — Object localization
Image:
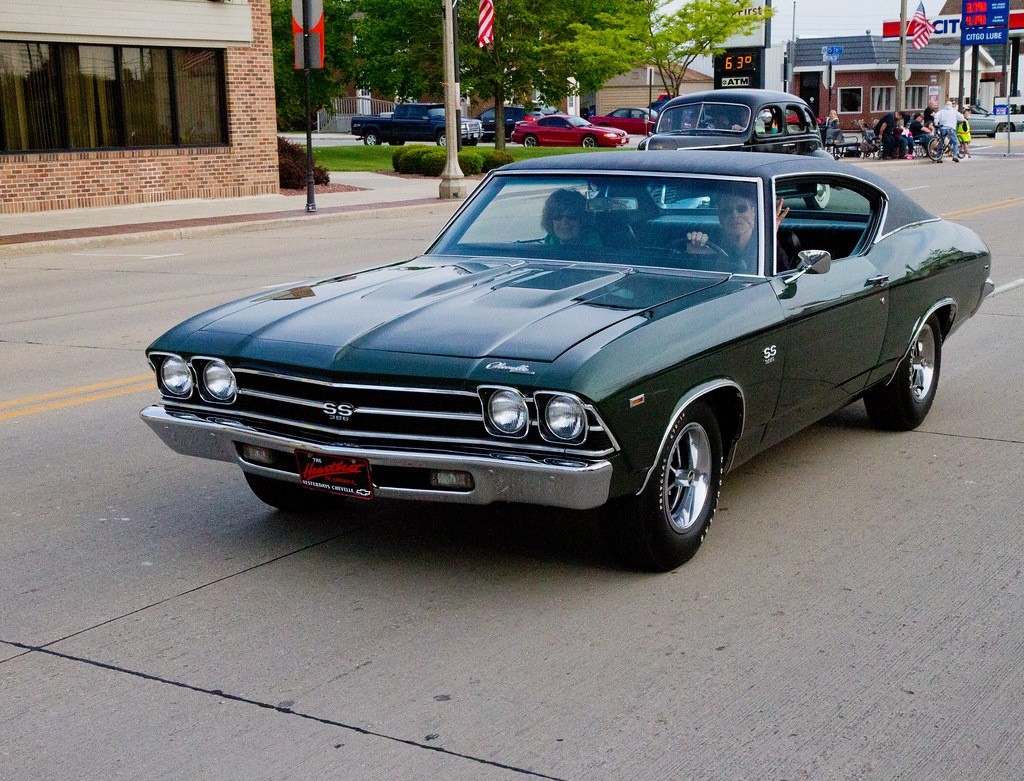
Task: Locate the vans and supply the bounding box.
[994,97,1024,133]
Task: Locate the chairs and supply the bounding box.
[592,221,645,246]
[863,130,886,158]
[906,123,925,158]
[776,228,802,272]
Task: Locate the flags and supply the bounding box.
[477,0,494,48]
[914,3,935,50]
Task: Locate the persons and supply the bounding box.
[874,100,971,163]
[822,110,839,152]
[687,185,790,273]
[540,189,602,247]
[732,110,752,131]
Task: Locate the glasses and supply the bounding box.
[550,210,580,222]
[718,204,753,215]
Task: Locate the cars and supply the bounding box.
[636,88,836,210]
[141,153,995,574]
[588,107,690,137]
[509,113,631,148]
[473,105,564,144]
[647,92,714,130]
[962,104,1024,138]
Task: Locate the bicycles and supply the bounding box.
[927,124,967,161]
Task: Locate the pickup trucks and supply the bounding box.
[351,101,484,147]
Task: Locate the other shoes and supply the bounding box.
[937,160,943,163]
[898,155,907,159]
[952,157,959,162]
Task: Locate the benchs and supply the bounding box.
[643,219,865,262]
[824,136,860,160]
